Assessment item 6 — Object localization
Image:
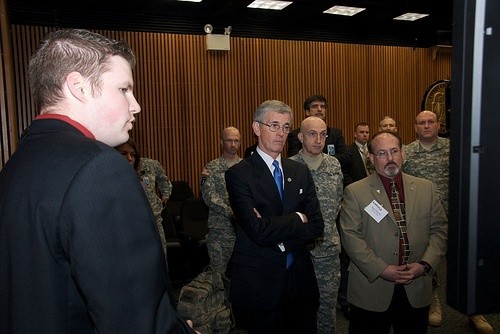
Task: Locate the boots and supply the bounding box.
[427,287,443,327]
[469,313,494,334]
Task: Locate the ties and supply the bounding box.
[389,178,408,268]
[361,145,367,169]
[272,159,293,269]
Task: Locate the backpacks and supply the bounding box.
[177,264,237,334]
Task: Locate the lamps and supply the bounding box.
[203,24,232,52]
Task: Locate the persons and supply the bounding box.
[225,100,323,334]
[0,28,205,333]
[135,157,172,272]
[200,126,245,273]
[285,116,345,334]
[287,95,348,168]
[339,130,446,334]
[379,116,398,134]
[401,110,494,333]
[343,121,374,185]
[116,138,142,172]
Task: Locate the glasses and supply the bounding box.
[309,104,328,111]
[256,119,293,134]
[374,148,403,159]
[306,130,329,139]
[223,137,241,145]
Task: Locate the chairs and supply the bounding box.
[157,180,211,286]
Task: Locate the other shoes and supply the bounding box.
[337,294,351,320]
